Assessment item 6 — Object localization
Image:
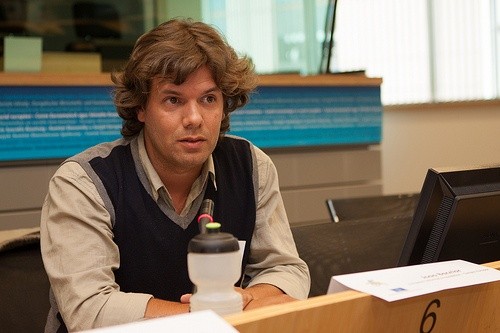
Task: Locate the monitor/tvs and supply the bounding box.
[397,166,500,264]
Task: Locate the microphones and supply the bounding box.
[197,198,215,233]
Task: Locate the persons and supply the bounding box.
[39,15,311,332]
